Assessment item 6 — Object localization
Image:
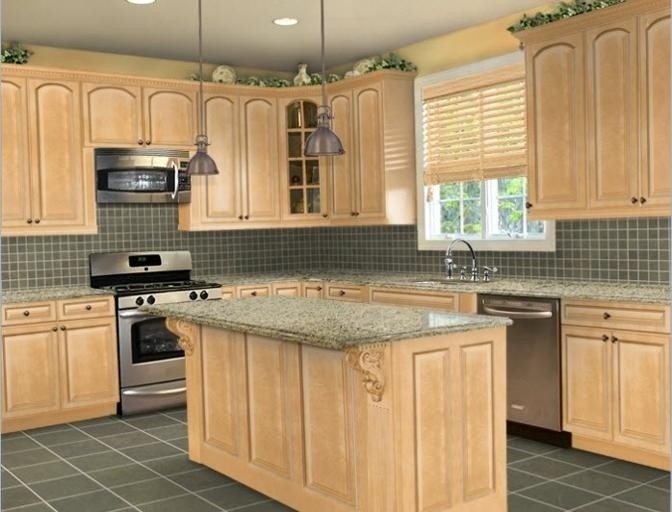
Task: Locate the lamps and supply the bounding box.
[303,1,345,156]
[188,1,221,176]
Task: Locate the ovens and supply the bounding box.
[118,309,188,416]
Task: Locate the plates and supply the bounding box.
[211,64,237,84]
[352,58,376,77]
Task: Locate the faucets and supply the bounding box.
[446,238,478,279]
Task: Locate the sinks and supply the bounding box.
[412,278,495,287]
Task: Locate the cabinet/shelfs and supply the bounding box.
[178,80,280,232]
[585,1,671,219]
[562,304,671,471]
[329,70,416,227]
[278,88,328,228]
[0,295,121,436]
[512,13,585,221]
[0,64,97,237]
[84,73,199,152]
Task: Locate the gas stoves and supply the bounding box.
[91,281,222,310]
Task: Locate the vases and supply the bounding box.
[294,63,312,85]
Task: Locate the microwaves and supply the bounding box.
[94,148,192,205]
